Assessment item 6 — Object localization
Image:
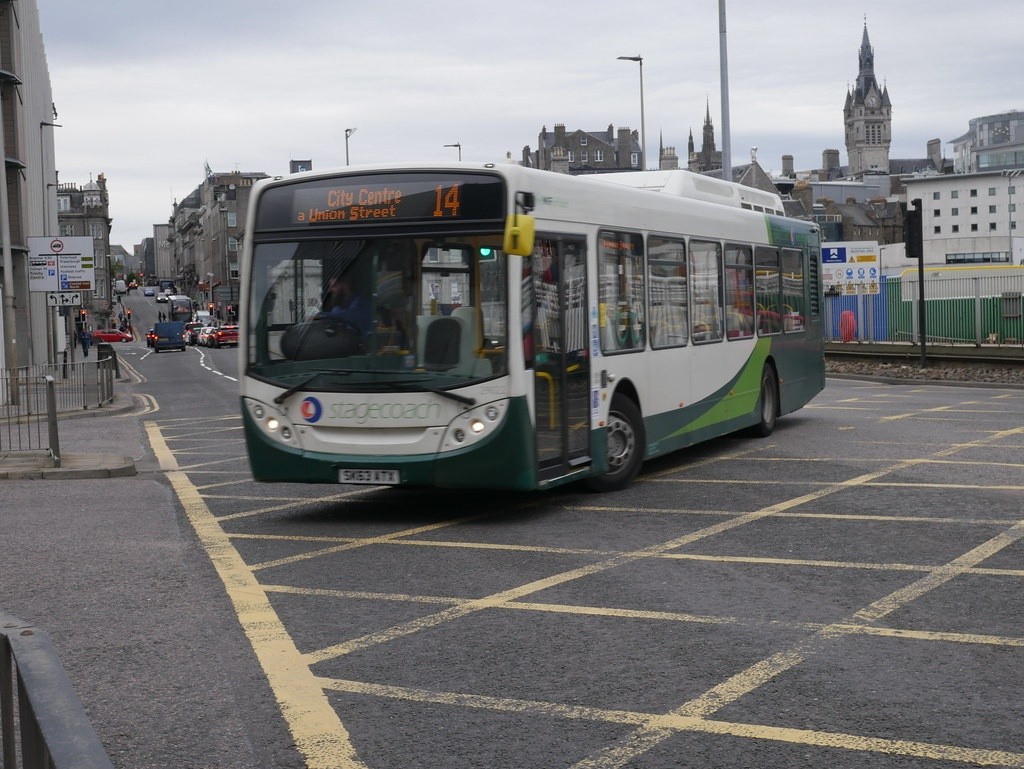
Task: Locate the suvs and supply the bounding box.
[167,294,192,322]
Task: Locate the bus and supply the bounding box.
[235,159,829,516]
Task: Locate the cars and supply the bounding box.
[128,282,139,289]
[144,310,240,354]
[93,329,133,344]
[163,288,175,296]
[155,292,169,304]
[144,288,156,296]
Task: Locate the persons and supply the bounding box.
[80,329,92,357]
[654,311,710,337]
[314,276,373,340]
[111,311,127,332]
[158,311,166,322]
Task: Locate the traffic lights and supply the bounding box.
[209,303,214,316]
[228,307,235,318]
[478,248,498,264]
[81,310,86,323]
[127,310,131,319]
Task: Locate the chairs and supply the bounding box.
[404,299,793,373]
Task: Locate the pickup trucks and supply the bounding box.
[158,278,178,296]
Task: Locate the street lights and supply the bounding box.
[617,54,647,171]
[344,125,358,166]
[443,142,462,162]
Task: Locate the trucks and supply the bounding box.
[114,280,128,294]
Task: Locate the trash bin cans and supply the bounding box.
[97,342,112,368]
